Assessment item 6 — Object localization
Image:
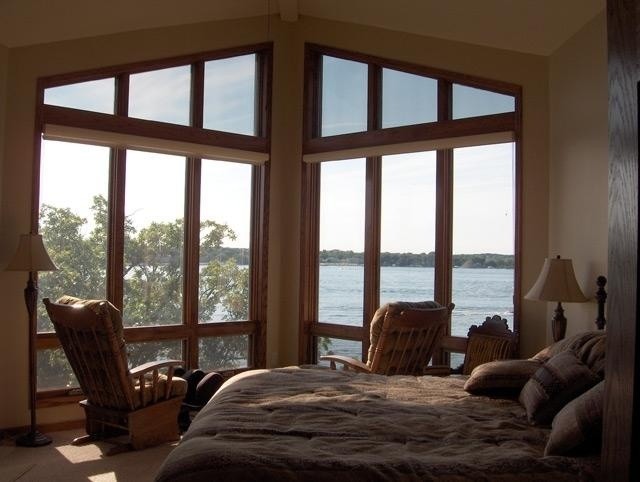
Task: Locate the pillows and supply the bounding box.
[544,380,605,457]
[463,358,543,395]
[518,350,602,426]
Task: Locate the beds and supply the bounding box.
[152,330,607,482]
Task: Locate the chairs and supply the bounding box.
[320,300,456,376]
[462,315,519,376]
[43,294,188,457]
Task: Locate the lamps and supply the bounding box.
[523,254,590,342]
[0,231,58,447]
